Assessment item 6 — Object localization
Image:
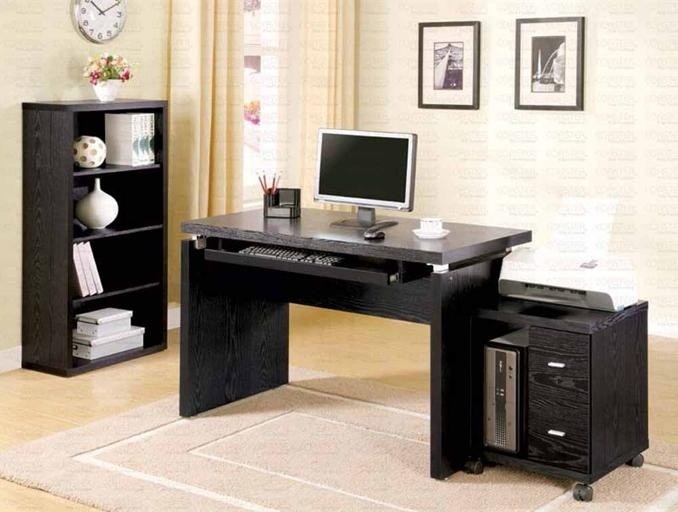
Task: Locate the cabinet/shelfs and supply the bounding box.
[20,99,170,378]
[466,293,652,500]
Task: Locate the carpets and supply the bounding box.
[0,382,678,512]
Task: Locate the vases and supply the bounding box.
[91,76,124,104]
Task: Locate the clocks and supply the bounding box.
[68,0,131,45]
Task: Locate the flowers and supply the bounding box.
[79,52,134,85]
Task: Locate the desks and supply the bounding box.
[176,206,533,482]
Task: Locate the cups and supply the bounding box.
[420,218,444,235]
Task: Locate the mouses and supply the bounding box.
[362,228,387,241]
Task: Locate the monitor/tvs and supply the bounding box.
[312,128,417,230]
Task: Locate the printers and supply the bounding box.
[499,244,639,314]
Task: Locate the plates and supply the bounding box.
[413,229,450,240]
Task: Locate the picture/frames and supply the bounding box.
[416,19,483,111]
[514,15,587,113]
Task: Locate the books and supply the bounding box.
[104,111,156,167]
[72,241,104,298]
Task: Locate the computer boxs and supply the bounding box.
[483,325,530,457]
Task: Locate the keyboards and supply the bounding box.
[236,241,342,268]
